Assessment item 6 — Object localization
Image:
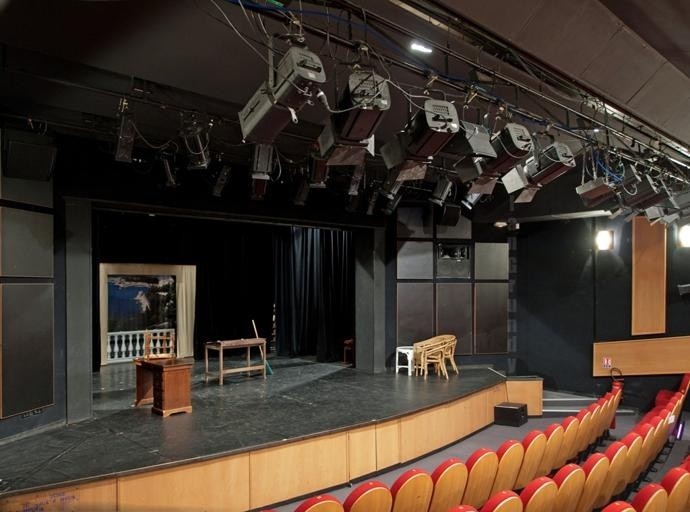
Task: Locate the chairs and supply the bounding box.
[444,372,690,512]
[343,480,392,512]
[390,469,434,512]
[395,333,459,381]
[430,458,469,511]
[294,494,344,512]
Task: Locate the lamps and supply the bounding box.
[117,2,690,226]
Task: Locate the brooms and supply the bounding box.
[252,319,273,375]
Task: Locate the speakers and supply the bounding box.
[438,202,461,226]
[5,140,56,181]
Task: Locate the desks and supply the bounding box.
[133,358,194,417]
[202,338,267,386]
